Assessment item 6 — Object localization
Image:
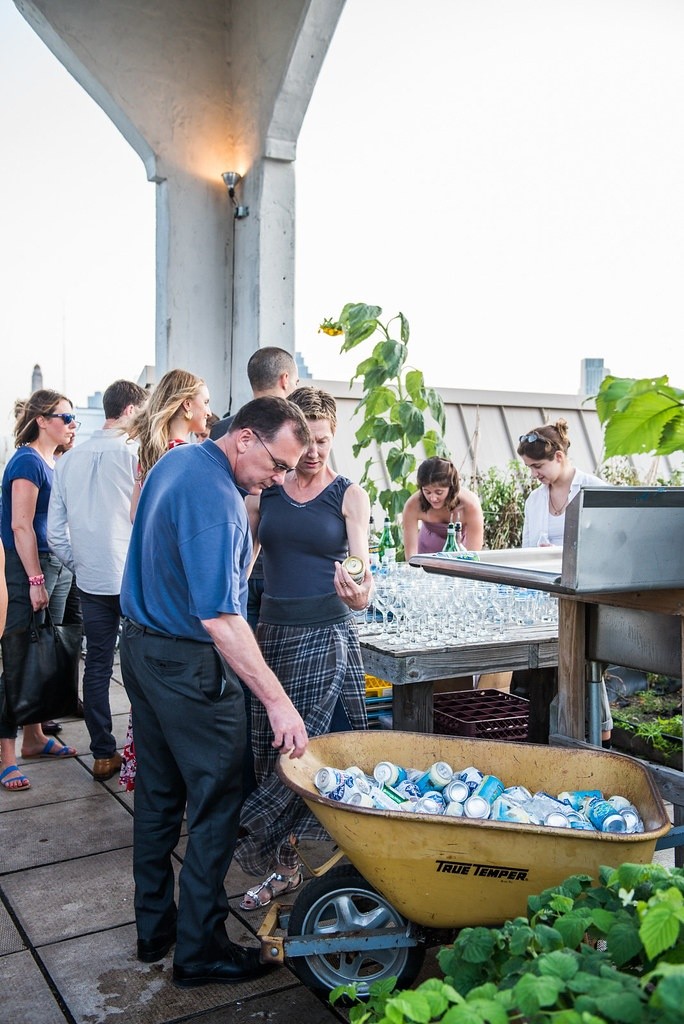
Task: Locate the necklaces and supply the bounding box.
[549,494,568,515]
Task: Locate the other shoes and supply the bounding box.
[43,720,62,734]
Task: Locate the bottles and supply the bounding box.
[537,530,551,547]
[455,512,467,551]
[442,512,460,552]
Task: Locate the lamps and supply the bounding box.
[221,171,248,218]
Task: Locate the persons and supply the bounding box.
[194,412,220,444]
[402,456,484,563]
[0,389,82,792]
[46,378,152,782]
[235,389,377,910]
[516,419,614,751]
[119,394,308,990]
[208,347,299,636]
[119,370,214,822]
[0,533,9,640]
[42,429,82,736]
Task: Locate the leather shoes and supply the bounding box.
[93,751,123,781]
[137,914,177,962]
[170,939,282,989]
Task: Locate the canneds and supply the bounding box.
[340,555,365,585]
[431,552,480,562]
[313,761,639,834]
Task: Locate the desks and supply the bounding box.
[355,609,561,734]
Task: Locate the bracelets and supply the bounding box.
[349,603,368,611]
[29,574,45,585]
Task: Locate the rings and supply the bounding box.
[341,582,347,588]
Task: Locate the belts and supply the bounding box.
[40,552,52,560]
[126,618,166,638]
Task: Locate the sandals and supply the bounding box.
[240,866,303,910]
[22,737,79,759]
[0,765,31,791]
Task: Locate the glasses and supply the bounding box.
[41,412,75,424]
[240,425,296,474]
[519,433,539,444]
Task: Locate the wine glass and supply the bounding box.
[358,562,558,650]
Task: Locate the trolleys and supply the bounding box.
[256,728,684,1008]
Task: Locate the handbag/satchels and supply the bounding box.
[0,605,84,728]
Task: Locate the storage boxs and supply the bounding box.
[434,689,530,742]
[365,674,392,698]
